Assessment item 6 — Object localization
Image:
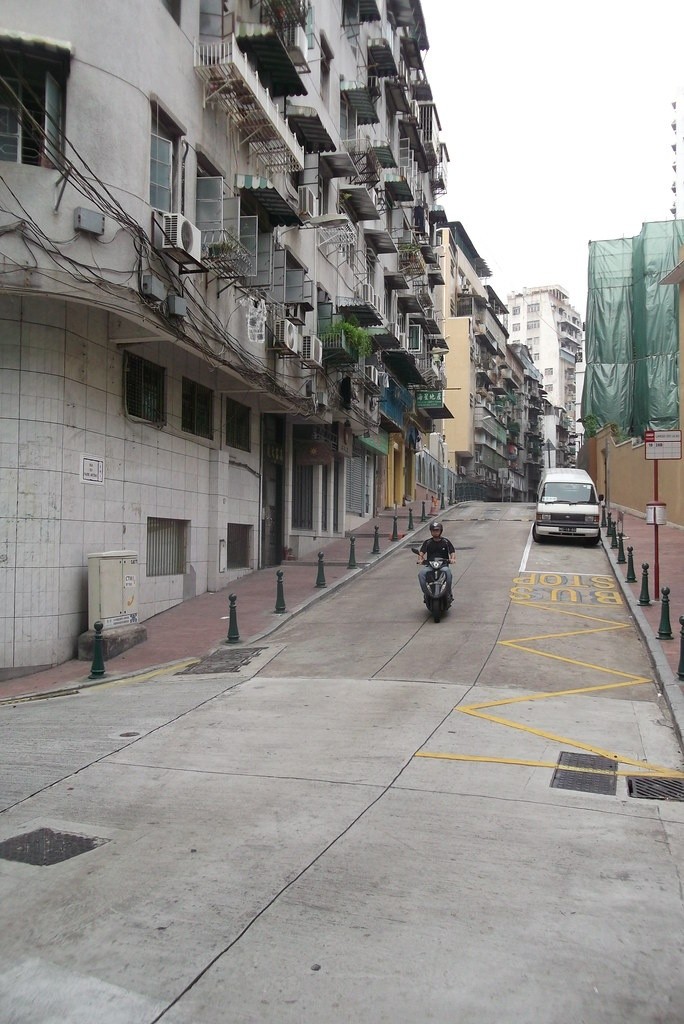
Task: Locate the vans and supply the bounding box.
[532,467,604,546]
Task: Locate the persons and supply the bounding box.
[417,522,456,603]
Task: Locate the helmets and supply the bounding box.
[430,523,443,530]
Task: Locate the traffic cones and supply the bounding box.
[428,496,438,516]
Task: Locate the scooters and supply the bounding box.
[411,547,455,623]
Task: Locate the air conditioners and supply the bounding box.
[368,76,380,95]
[459,466,466,475]
[300,335,323,367]
[290,305,305,325]
[365,365,378,386]
[409,100,419,125]
[273,320,298,355]
[363,284,381,315]
[284,23,308,63]
[390,322,399,340]
[298,186,317,221]
[399,333,408,350]
[161,213,201,264]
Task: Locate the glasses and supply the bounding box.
[433,529,439,531]
